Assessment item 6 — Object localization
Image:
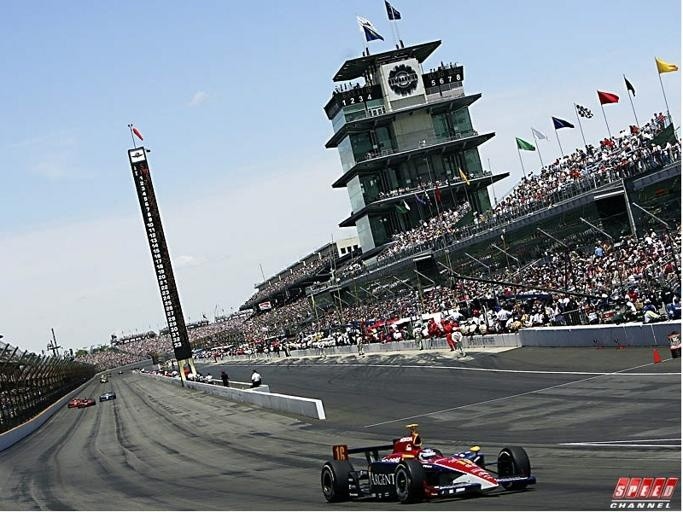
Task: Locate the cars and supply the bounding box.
[68,392,115,409]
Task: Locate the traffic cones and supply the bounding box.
[650,347,661,365]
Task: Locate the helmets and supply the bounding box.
[419,448,437,465]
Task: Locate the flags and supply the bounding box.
[624,77,635,97]
[356,16,385,42]
[385,0,401,20]
[655,57,678,74]
[597,90,619,105]
[575,103,594,119]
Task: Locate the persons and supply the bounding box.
[70,108,680,388]
